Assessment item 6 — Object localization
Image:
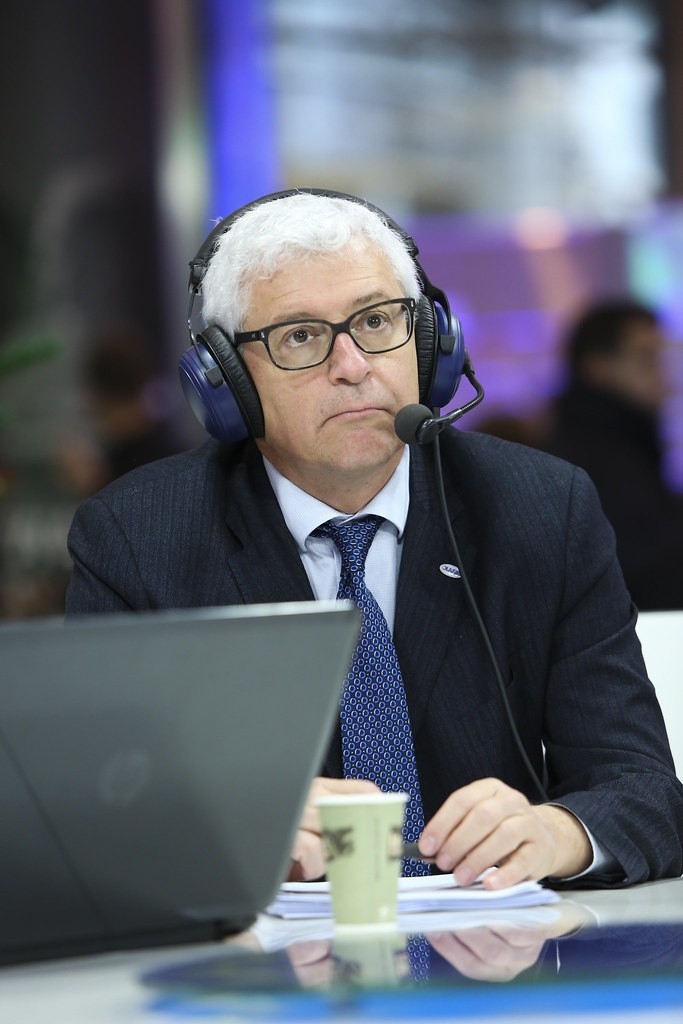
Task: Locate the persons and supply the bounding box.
[64,185,682,890]
[506,293,682,612]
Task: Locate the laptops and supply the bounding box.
[0,600,364,965]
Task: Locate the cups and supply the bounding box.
[315,794,410,922]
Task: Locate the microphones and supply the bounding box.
[394,358,484,447]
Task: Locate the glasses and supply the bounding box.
[235,298,417,370]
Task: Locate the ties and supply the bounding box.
[310,516,432,877]
[404,933,431,989]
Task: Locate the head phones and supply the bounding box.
[178,188,468,445]
